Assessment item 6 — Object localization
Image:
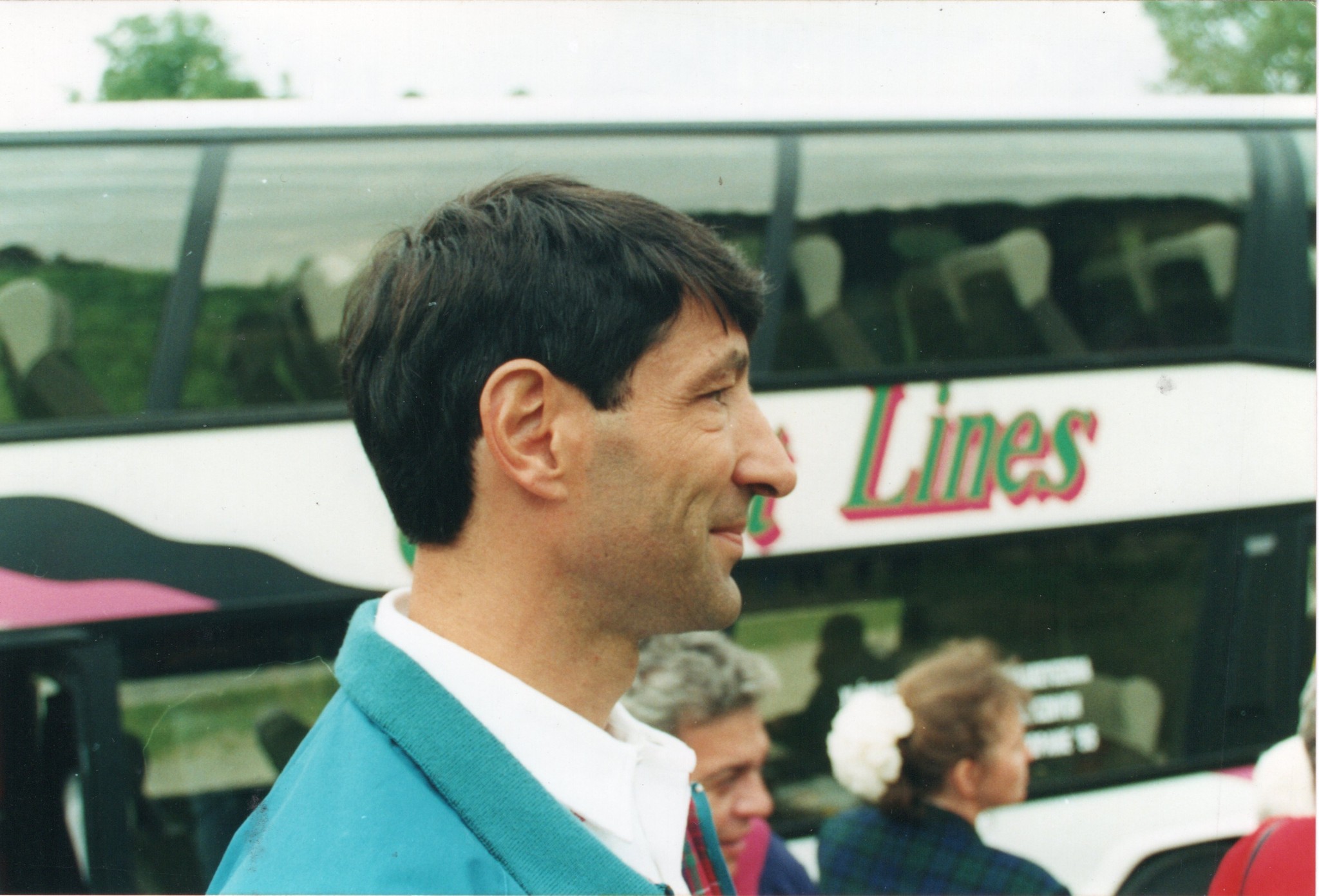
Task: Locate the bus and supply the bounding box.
[0,88,1317,896]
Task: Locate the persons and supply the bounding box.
[500,631,776,896]
[1187,649,1317,896]
[196,173,796,896]
[820,635,1071,896]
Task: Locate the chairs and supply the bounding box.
[0,226,1319,889]
